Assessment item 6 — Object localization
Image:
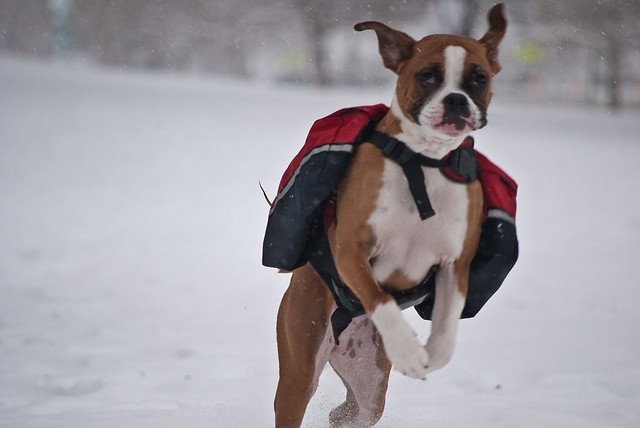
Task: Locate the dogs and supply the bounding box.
[259,2,508,428]
